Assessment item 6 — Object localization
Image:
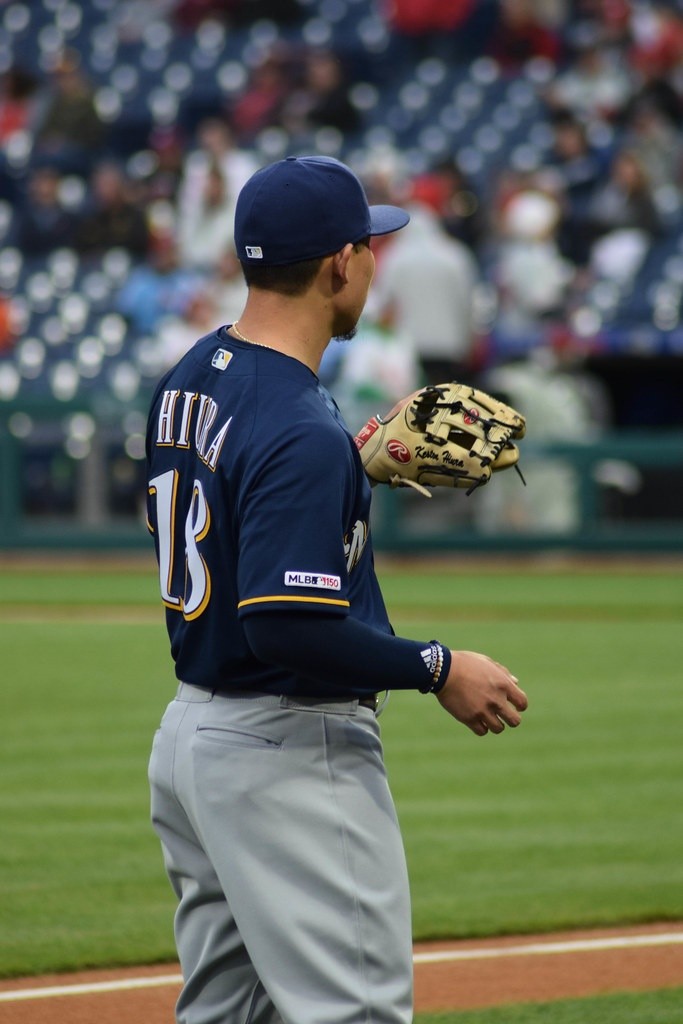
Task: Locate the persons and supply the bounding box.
[0,4,683,538]
[135,156,535,1024]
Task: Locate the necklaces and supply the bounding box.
[233,319,273,352]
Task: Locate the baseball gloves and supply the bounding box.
[350,381,529,499]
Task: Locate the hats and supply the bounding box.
[233,156,411,267]
